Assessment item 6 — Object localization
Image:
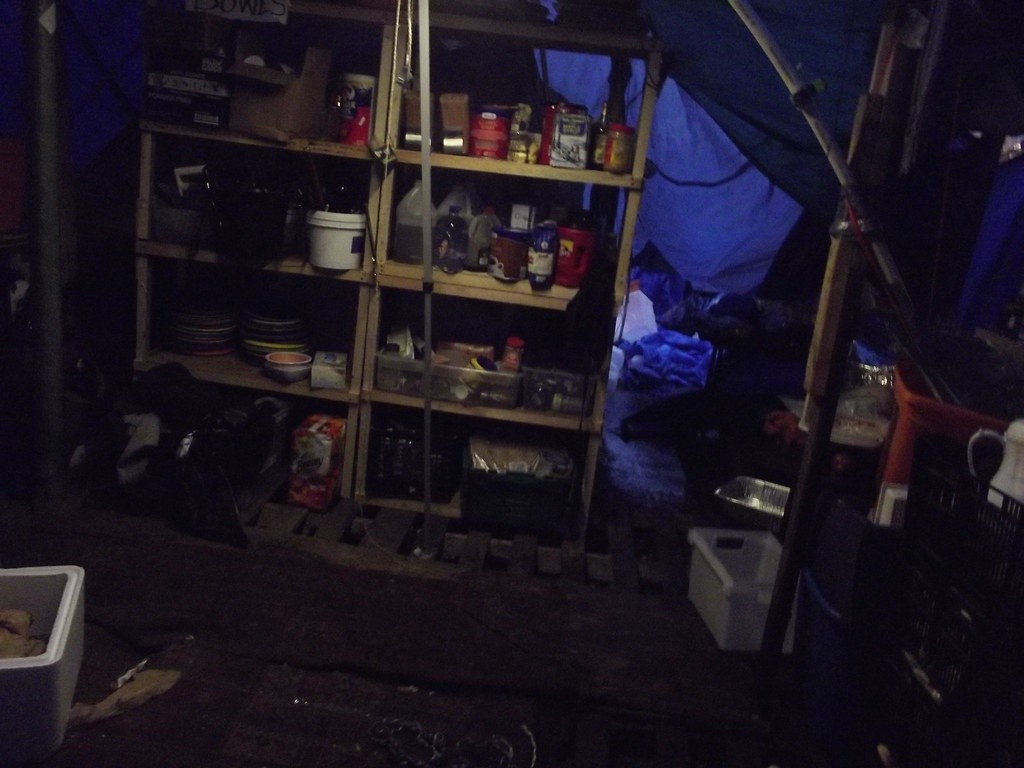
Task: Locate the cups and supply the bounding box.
[553,225,600,289]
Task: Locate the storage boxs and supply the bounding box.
[520,362,598,419]
[713,475,793,534]
[229,29,334,143]
[311,348,349,392]
[881,432,1024,768]
[457,423,583,541]
[287,412,346,510]
[144,88,228,132]
[685,527,801,656]
[373,347,523,409]
[0,565,86,768]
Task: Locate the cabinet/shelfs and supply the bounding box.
[352,0,668,545]
[127,3,401,506]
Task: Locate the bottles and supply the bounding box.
[587,101,611,171]
[392,179,503,274]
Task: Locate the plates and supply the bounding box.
[165,306,313,363]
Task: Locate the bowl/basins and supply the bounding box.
[265,351,312,381]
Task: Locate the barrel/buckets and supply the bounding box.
[306,208,367,270]
